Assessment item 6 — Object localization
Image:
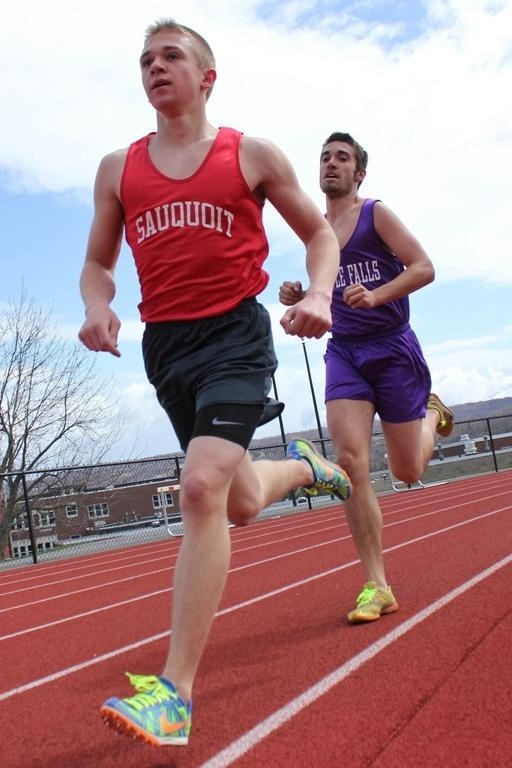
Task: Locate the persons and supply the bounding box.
[280,132,456,624]
[80,19,354,748]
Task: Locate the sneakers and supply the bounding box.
[287,438,352,500]
[347,581,398,624]
[100,671,192,747]
[427,393,454,437]
